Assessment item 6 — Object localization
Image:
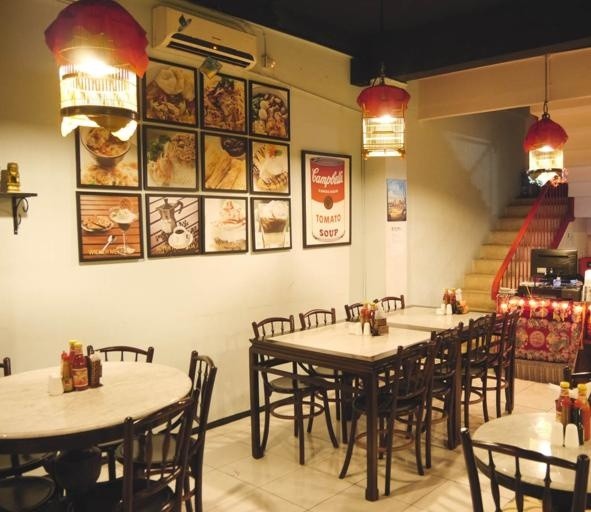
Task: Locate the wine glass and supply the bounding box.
[108,207,137,255]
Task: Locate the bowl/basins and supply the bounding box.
[81,131,131,167]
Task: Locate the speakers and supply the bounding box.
[350,58,378,86]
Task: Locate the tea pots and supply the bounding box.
[156,198,184,233]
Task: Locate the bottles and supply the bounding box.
[360,300,376,336]
[557,381,591,446]
[443,287,456,314]
[60,340,89,393]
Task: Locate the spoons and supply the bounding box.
[99,234,116,255]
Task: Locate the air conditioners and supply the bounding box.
[151,3,258,71]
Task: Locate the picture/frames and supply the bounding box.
[75,121,143,191]
[249,79,291,142]
[198,69,249,135]
[250,138,292,196]
[141,70,198,129]
[202,194,249,254]
[249,196,292,253]
[145,192,205,259]
[200,130,249,194]
[301,150,352,248]
[75,190,146,262]
[141,123,200,193]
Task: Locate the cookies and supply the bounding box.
[82,215,110,230]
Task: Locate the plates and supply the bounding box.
[168,234,193,250]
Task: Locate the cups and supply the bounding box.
[172,226,191,243]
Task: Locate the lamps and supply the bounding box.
[522,51,568,188]
[44,0,149,143]
[356,0,410,161]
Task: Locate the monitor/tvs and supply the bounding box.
[530,248,578,283]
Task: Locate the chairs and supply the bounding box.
[0,343,219,512]
[458,363,591,512]
[246,295,523,503]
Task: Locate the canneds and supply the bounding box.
[310,157,345,242]
